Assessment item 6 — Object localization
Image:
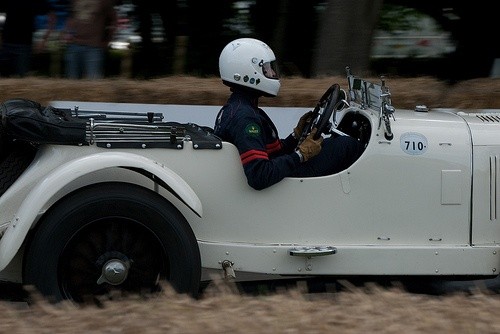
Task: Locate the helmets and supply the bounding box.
[219,38,281,96]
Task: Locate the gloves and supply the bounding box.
[299,127,324,162]
[293,111,313,137]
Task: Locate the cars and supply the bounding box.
[0,64,499,304]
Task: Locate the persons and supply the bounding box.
[214,37,366,191]
